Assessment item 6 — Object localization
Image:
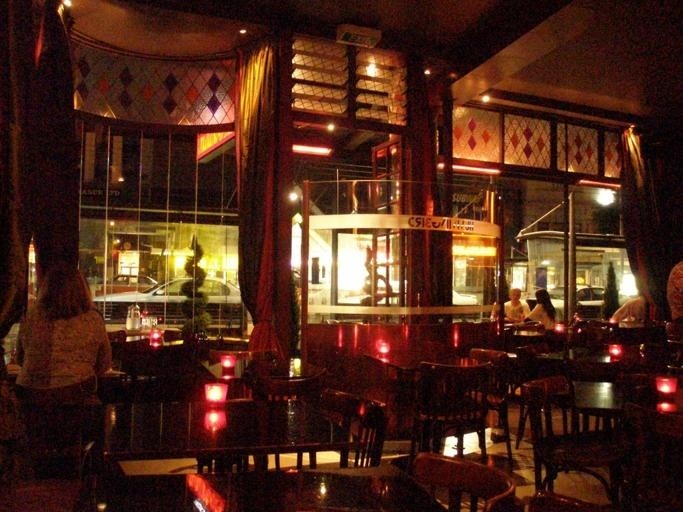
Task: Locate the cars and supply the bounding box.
[504,285,631,318]
[338,279,478,305]
[93,273,241,302]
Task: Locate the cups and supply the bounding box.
[221,355,237,375]
[143,317,157,331]
[150,333,163,347]
[607,343,625,365]
[372,464,400,499]
[555,324,565,335]
[654,375,678,398]
[202,409,226,429]
[202,383,228,409]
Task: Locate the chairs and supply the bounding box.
[1,320,682,511]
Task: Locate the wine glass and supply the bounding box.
[375,339,393,365]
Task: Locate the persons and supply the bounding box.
[16,261,112,414]
[504,289,557,330]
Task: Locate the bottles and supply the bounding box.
[124,301,141,332]
